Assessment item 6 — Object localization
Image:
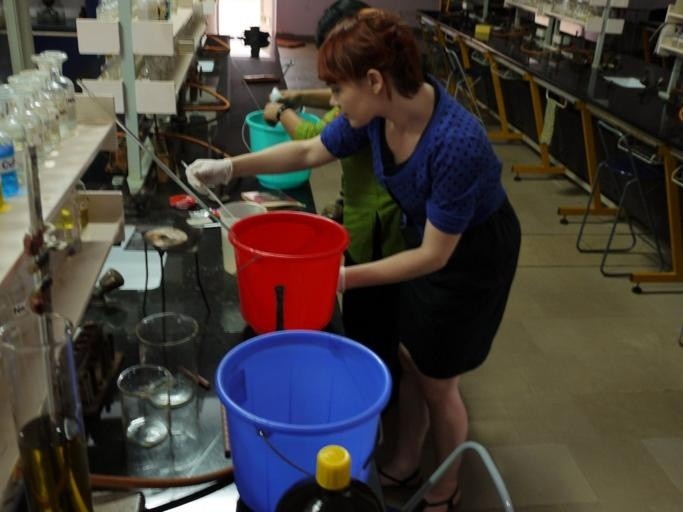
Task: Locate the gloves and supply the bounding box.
[186,157,234,198]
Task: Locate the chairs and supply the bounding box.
[443,46,488,126]
[576,122,665,278]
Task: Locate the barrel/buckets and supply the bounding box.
[240,110,326,192]
[227,209,352,337]
[213,328,395,512]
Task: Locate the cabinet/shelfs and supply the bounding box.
[72,1,209,200]
[2,83,130,508]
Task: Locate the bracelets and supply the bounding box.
[276,103,290,121]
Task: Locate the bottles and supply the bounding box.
[94,0,204,83]
[0,307,99,512]
[0,48,81,215]
[272,442,384,512]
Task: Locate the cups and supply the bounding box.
[116,362,173,449]
[216,200,272,278]
[134,312,202,409]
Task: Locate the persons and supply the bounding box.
[184,13,522,511]
[262,0,408,440]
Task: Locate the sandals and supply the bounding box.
[380,467,423,490]
[413,484,464,512]
